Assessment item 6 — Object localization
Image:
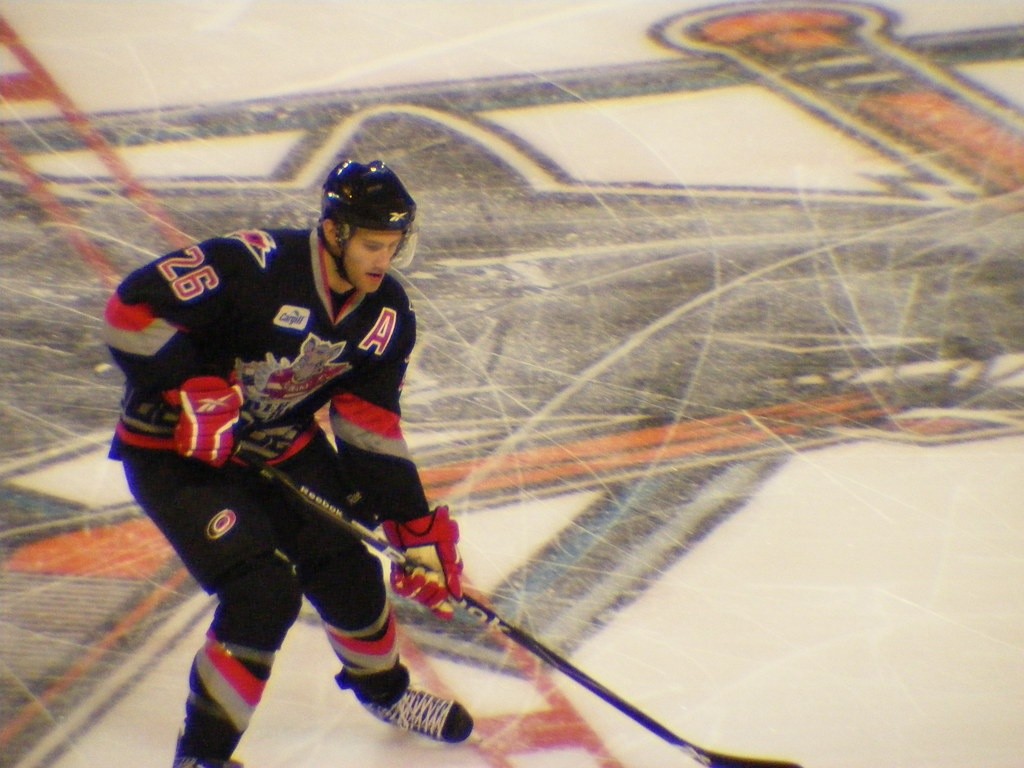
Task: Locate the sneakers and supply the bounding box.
[171,719,233,768]
[335,669,474,750]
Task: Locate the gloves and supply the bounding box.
[383,504,464,620]
[162,370,244,469]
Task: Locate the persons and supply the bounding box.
[102,161,474,768]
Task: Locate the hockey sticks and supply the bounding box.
[235,443,803,768]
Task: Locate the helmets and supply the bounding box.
[322,159,417,231]
